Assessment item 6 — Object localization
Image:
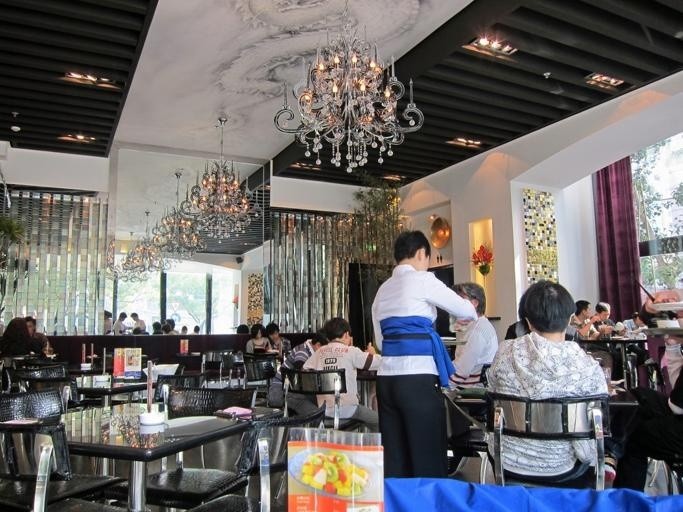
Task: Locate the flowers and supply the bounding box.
[472,244,492,267]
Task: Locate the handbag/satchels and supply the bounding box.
[644,457,680,495]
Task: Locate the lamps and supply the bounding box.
[109,116,264,283]
[274,0,425,175]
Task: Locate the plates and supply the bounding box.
[655,321,681,329]
[439,334,457,340]
[652,300,683,311]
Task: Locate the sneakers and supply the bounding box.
[448,455,468,477]
[604,457,617,489]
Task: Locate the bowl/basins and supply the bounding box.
[138,424,165,435]
[80,363,91,367]
[139,412,164,425]
[80,368,91,371]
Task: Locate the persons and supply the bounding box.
[486,279,609,489]
[572,298,649,383]
[237,322,291,359]
[371,230,478,480]
[0,317,47,362]
[25,316,53,356]
[302,318,378,433]
[505,294,578,344]
[104,310,201,336]
[267,329,327,416]
[604,336,683,493]
[444,282,499,477]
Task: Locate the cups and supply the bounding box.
[76,382,109,387]
[100,393,112,418]
[75,374,108,382]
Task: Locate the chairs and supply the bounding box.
[0,321,683,512]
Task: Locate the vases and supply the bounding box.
[478,264,490,275]
